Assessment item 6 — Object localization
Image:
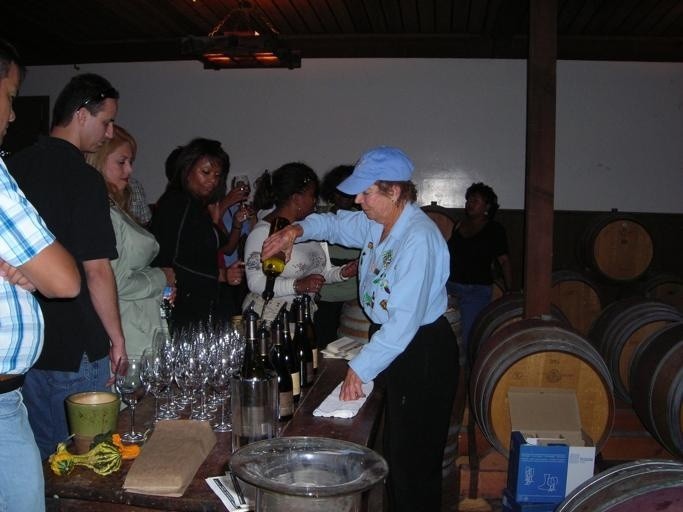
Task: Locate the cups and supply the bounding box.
[65,392,122,456]
[229,373,279,454]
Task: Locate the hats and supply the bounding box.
[334,144,417,198]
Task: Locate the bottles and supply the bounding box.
[255,169,273,211]
[238,292,320,448]
[259,216,292,301]
[159,284,174,319]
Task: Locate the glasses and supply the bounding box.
[77,85,120,113]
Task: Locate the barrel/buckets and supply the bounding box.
[420,200,456,242]
[335,297,374,344]
[441,208,683,512]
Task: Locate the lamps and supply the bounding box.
[184,0,303,72]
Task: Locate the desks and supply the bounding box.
[39,354,385,511]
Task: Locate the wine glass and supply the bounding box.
[114,315,249,443]
[232,174,251,212]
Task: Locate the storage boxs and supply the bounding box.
[502,383,598,506]
[496,489,557,510]
